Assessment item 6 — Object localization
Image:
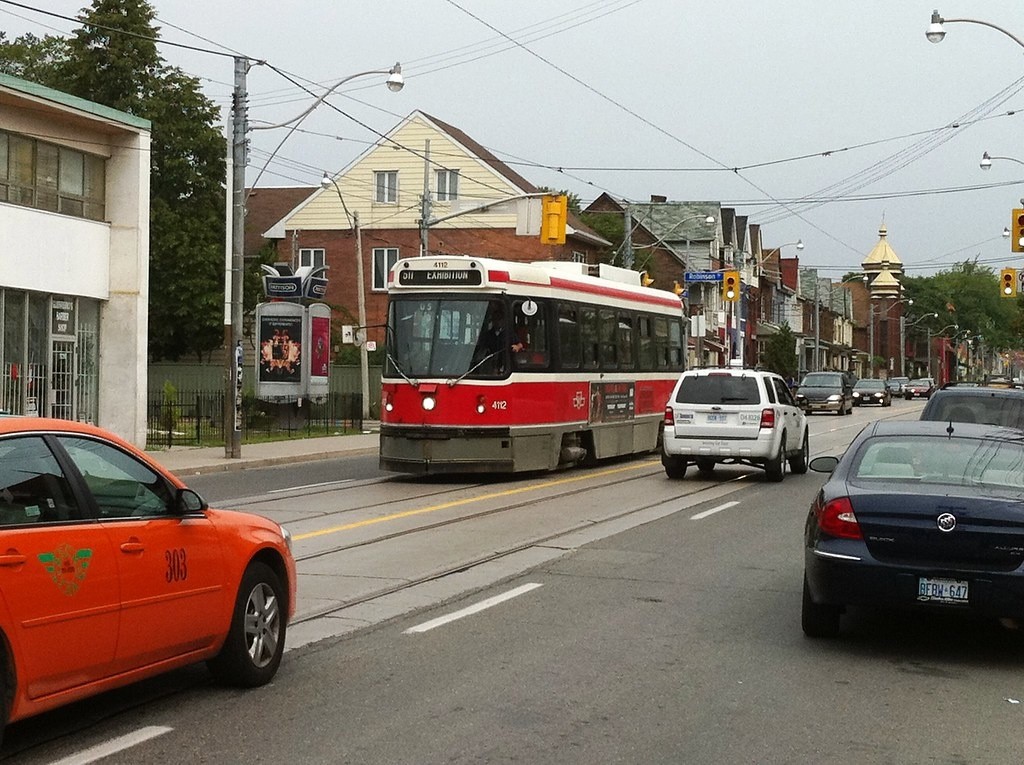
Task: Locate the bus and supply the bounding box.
[353,255,690,475]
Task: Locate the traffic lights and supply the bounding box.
[676,288,687,297]
[723,272,741,302]
[645,275,655,287]
[1000,269,1017,298]
[1012,209,1024,253]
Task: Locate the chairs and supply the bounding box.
[877,445,911,464]
[6,459,53,521]
[982,469,1024,485]
[869,461,912,477]
[948,406,976,421]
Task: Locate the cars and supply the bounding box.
[0,415,299,731]
[661,368,1024,651]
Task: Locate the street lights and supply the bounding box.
[624,207,973,378]
[320,168,371,423]
[225,58,410,463]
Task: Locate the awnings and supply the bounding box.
[702,338,728,353]
[756,321,780,336]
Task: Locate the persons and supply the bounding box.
[481,311,522,374]
